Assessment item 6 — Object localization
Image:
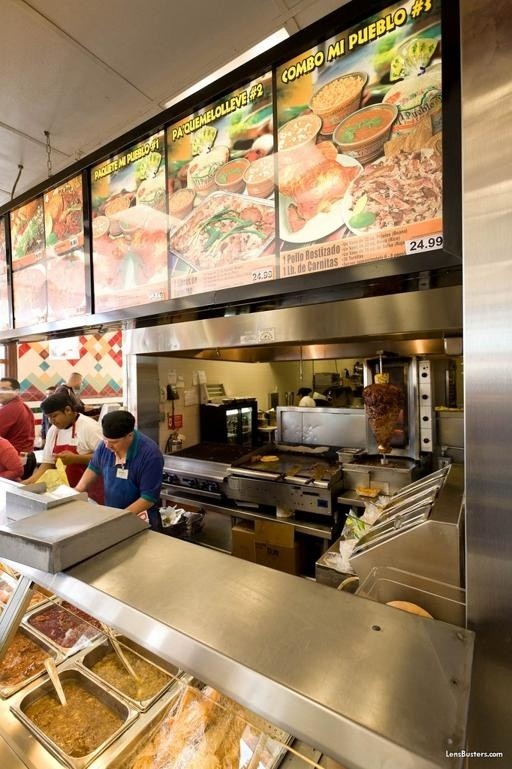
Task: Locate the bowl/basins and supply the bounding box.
[92,194,143,238]
[166,70,444,242]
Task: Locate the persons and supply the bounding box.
[70,410,166,534]
[18,391,106,507]
[0,377,37,481]
[296,387,316,407]
[0,435,25,484]
[39,384,87,449]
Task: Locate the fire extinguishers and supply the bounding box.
[164,431,181,452]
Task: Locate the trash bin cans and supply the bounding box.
[163,510,204,545]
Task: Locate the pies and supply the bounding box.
[260,455,280,462]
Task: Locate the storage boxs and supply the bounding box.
[229,517,310,576]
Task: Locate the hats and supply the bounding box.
[39,392,71,416]
[296,387,312,396]
[101,410,136,439]
